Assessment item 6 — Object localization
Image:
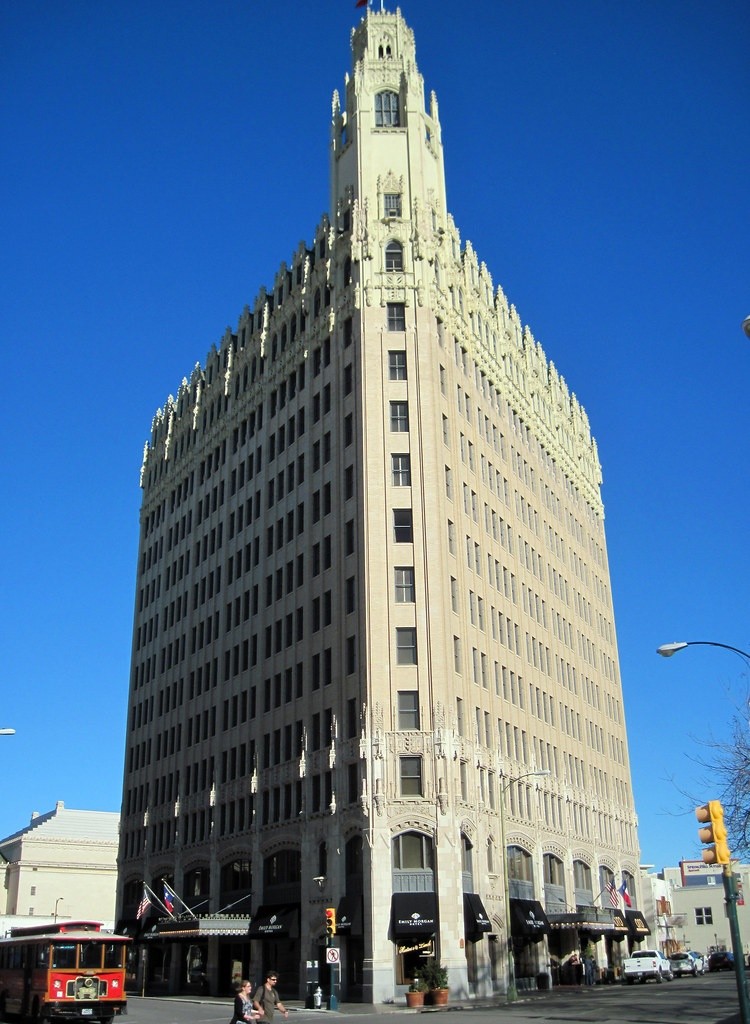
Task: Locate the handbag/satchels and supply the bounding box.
[252,985,265,1011]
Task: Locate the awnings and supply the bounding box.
[609,910,651,935]
[335,896,364,937]
[250,904,300,939]
[392,892,439,935]
[509,898,552,936]
[463,893,492,932]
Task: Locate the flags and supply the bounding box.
[618,878,632,908]
[163,884,175,912]
[604,876,619,908]
[137,885,153,920]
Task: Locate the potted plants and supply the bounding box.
[405,980,427,1007]
[424,960,448,1004]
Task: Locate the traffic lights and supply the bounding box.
[325,908,335,934]
[695,800,730,865]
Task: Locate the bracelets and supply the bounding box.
[283,1010,288,1014]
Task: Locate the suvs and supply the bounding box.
[669,951,704,978]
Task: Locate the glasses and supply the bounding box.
[271,979,277,981]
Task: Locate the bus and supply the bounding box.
[0,921,133,1024]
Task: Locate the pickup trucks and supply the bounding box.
[623,950,673,984]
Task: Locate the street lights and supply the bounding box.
[500,770,551,1000]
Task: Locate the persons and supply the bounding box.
[253,970,288,1024]
[38,955,49,968]
[229,980,265,1024]
[708,947,717,959]
[569,954,612,986]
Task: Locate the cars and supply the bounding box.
[708,952,735,972]
